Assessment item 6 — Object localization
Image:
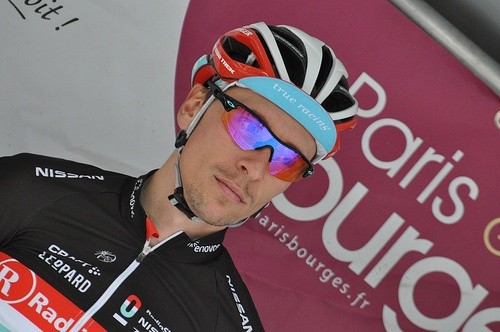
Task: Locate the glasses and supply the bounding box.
[210,81,314,182]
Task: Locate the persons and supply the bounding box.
[0,22,358,332]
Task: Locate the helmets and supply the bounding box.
[185,23,360,167]
